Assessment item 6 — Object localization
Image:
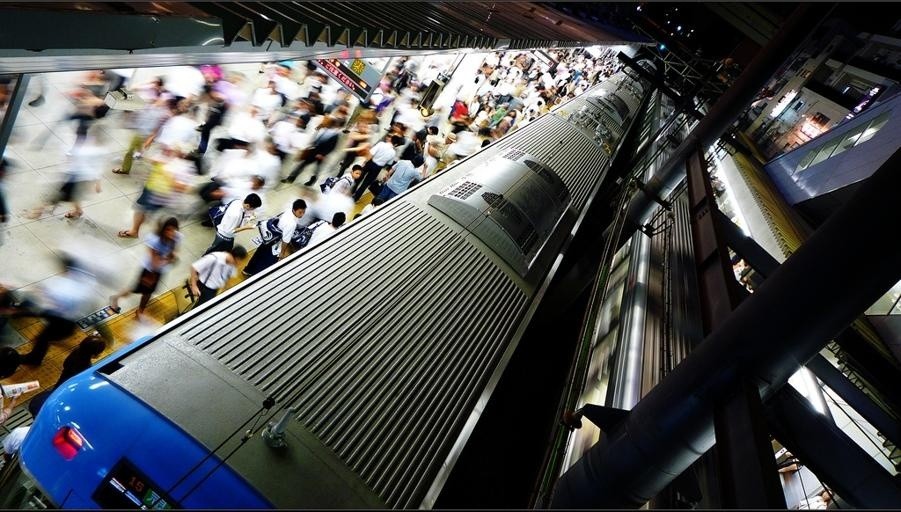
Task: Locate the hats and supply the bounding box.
[336,105,349,116]
[300,114,311,130]
[446,133,457,143]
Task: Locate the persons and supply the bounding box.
[0,59,314,477]
[315,45,621,250]
[796,489,834,510]
[777,452,803,476]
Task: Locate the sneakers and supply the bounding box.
[242,269,255,276]
[18,353,42,367]
[111,168,130,175]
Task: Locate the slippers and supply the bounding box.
[118,227,140,239]
[110,305,122,314]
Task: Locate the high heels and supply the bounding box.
[65,209,83,220]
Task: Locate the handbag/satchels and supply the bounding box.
[370,180,386,197]
[174,276,201,316]
[208,204,229,226]
[258,216,284,245]
[293,222,313,248]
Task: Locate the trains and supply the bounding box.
[536,81,715,509]
[0,58,658,510]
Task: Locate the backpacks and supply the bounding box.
[321,173,353,195]
